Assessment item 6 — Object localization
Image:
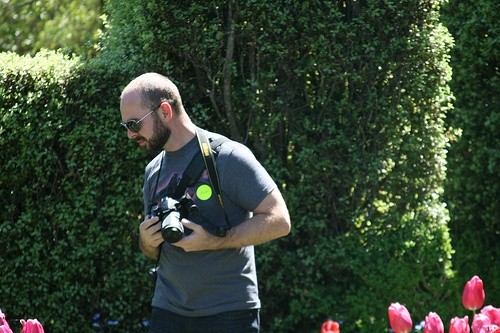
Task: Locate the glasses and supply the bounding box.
[120,102,169,133]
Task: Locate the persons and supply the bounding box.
[119,72,291,333]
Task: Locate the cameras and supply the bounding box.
[151,193,200,242]
